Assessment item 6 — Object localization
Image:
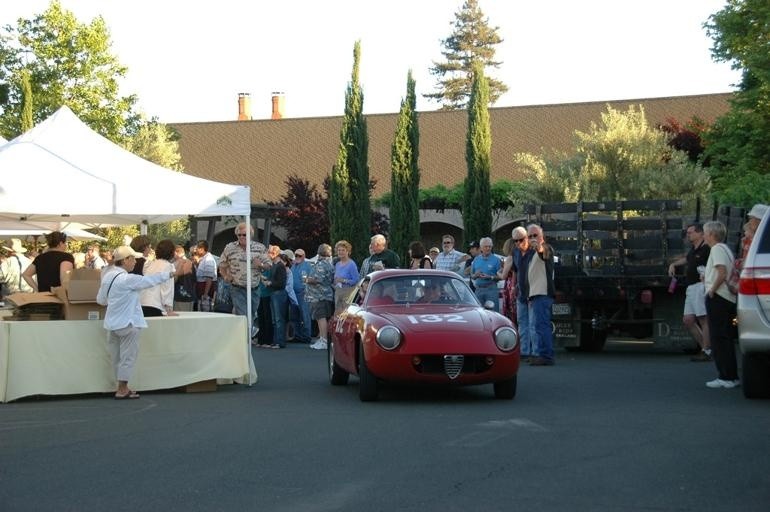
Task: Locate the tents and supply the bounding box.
[0,105,252,388]
[0,132,109,261]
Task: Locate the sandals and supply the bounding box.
[112,391,140,399]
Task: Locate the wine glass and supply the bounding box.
[302,270,307,281]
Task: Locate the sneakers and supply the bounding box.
[706,378,735,388]
[689,351,713,361]
[733,379,740,386]
[309,336,322,348]
[528,357,554,365]
[313,338,328,350]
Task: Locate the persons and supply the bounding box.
[190,238,220,312]
[96,246,182,399]
[367,234,401,275]
[286,248,314,343]
[171,244,195,313]
[256,267,273,348]
[334,240,360,311]
[357,281,394,307]
[407,241,440,271]
[703,222,741,389]
[511,226,538,359]
[497,238,518,329]
[261,245,288,349]
[667,224,713,362]
[519,224,556,365]
[128,235,152,277]
[738,202,766,271]
[304,244,333,349]
[140,240,179,317]
[219,222,272,335]
[469,237,501,313]
[279,253,299,306]
[413,282,460,305]
[432,235,472,277]
[464,241,482,294]
[0,238,36,298]
[358,244,372,280]
[21,232,75,293]
[79,246,114,270]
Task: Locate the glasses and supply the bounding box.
[444,242,450,244]
[238,234,246,236]
[528,233,537,238]
[295,255,302,257]
[515,237,526,242]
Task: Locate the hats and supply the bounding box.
[284,249,295,260]
[746,204,768,219]
[112,245,136,262]
[430,247,439,253]
[466,240,479,248]
[2,238,27,253]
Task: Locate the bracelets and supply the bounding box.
[491,276,495,281]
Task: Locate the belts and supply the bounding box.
[231,283,259,291]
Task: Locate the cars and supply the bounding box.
[321,267,521,403]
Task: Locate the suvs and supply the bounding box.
[734,205,770,400]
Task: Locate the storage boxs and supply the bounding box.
[64,268,102,301]
[50,287,107,321]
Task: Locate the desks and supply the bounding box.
[9,310,247,402]
